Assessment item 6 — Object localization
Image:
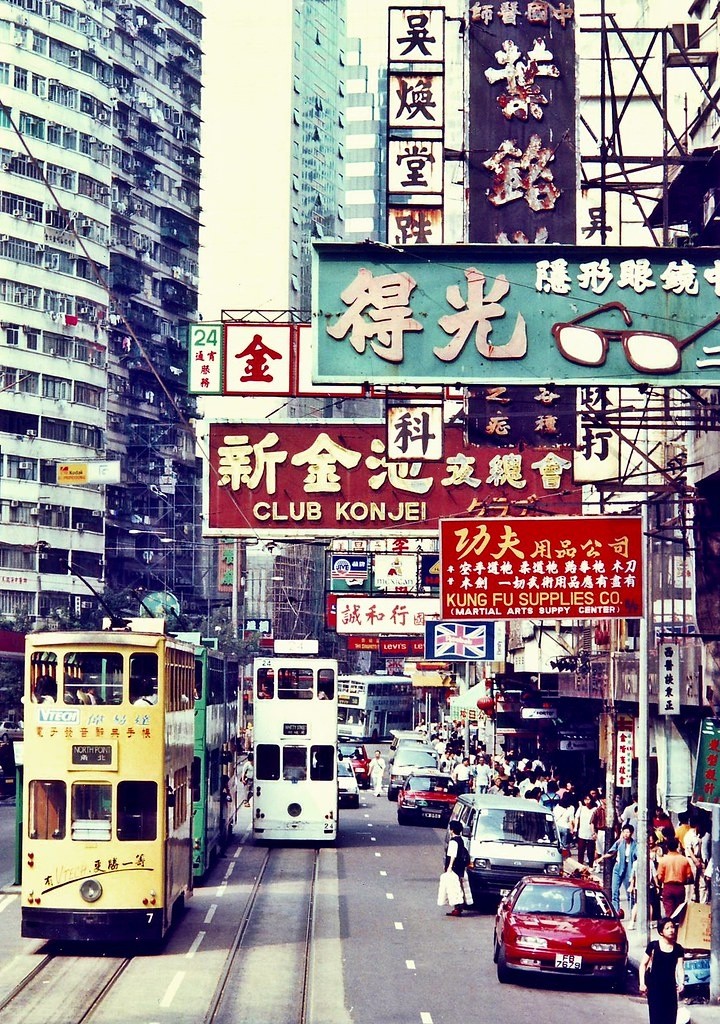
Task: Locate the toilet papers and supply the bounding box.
[446,869,464,907]
[436,870,451,907]
[462,870,474,905]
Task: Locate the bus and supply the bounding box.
[20,627,239,952]
[250,655,339,843]
[336,673,415,743]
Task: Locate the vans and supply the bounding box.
[385,728,433,747]
[442,792,564,915]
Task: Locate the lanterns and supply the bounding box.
[477,696,494,716]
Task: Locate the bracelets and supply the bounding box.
[682,983,684,986]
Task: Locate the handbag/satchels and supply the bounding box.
[463,846,470,867]
[437,872,448,907]
[240,776,248,785]
[447,871,463,906]
[644,941,655,986]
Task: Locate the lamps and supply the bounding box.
[550,654,592,676]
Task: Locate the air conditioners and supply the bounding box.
[77,523,84,530]
[31,507,40,515]
[14,19,112,87]
[41,553,48,559]
[92,511,100,516]
[45,505,52,510]
[19,462,32,470]
[0,111,111,369]
[59,506,65,511]
[27,429,37,436]
[99,560,107,565]
[11,500,19,507]
[666,19,718,65]
[82,602,92,609]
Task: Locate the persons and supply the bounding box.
[34,672,158,706]
[415,718,712,935]
[444,820,465,916]
[242,753,253,807]
[367,750,386,797]
[638,917,685,1024]
[259,683,272,699]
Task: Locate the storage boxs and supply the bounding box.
[671,901,711,985]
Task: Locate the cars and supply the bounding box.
[339,741,373,790]
[397,768,460,829]
[385,749,439,802]
[0,720,23,744]
[393,743,439,762]
[493,873,630,989]
[337,753,360,810]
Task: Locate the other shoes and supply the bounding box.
[244,804,250,807]
[377,794,380,797]
[628,923,635,930]
[446,908,461,917]
